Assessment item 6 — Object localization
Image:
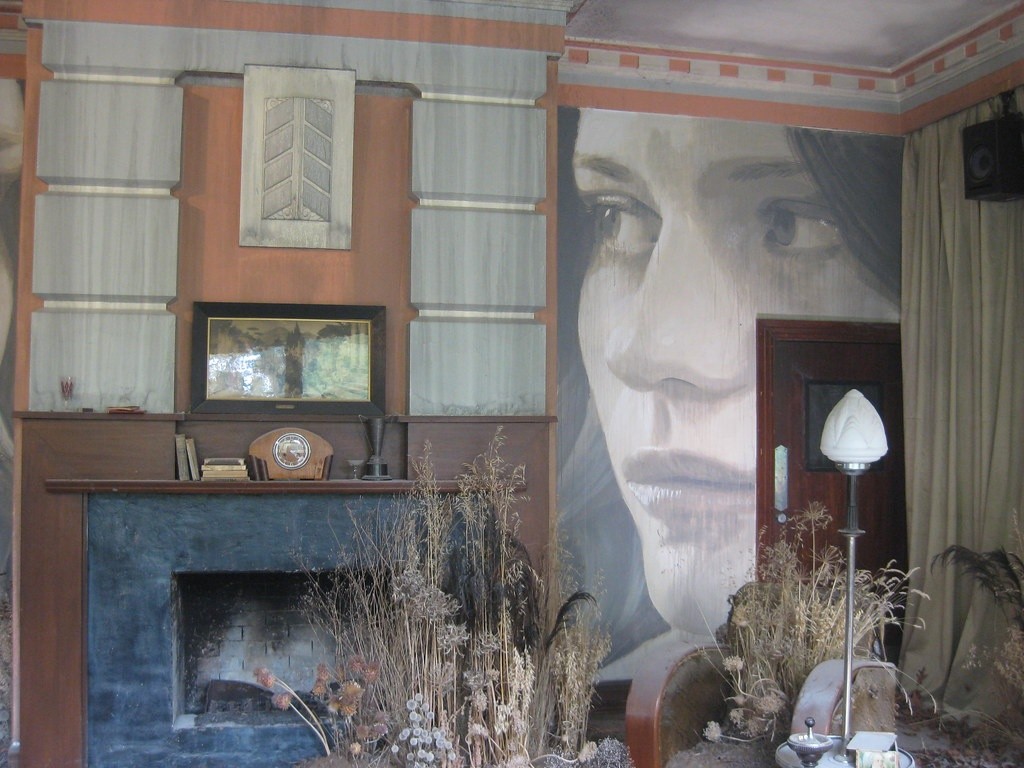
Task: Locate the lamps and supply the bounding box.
[821,386,888,758]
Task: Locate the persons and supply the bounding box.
[557,108,901,711]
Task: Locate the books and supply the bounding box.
[175,434,200,480]
[201,458,250,481]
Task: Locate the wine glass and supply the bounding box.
[60,376,73,412]
[346,459,365,480]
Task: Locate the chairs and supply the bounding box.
[616,578,892,767]
